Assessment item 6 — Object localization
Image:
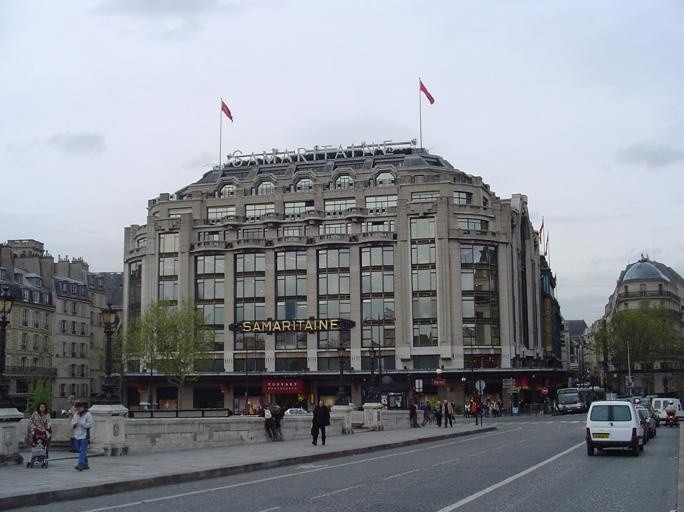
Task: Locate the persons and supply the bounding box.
[268,401,284,442]
[69,401,95,472]
[310,399,330,446]
[408,399,455,428]
[26,402,52,463]
[663,400,679,426]
[62,393,80,453]
[32,437,46,448]
[464,396,503,418]
[263,406,275,438]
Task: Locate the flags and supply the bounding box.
[222,101,234,123]
[538,222,544,243]
[421,83,435,105]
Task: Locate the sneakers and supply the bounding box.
[312,441,326,446]
[74,463,91,471]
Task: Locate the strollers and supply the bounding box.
[26,435,52,472]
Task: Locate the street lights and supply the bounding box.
[1,283,17,408]
[365,341,380,404]
[91,302,125,405]
[236,336,250,414]
[143,346,165,406]
[461,369,476,398]
[332,341,350,406]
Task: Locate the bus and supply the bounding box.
[554,386,606,414]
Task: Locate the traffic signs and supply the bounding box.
[502,378,520,394]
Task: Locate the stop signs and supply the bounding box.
[542,387,549,396]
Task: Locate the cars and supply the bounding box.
[627,390,684,439]
[283,406,309,416]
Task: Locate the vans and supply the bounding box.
[584,398,647,457]
